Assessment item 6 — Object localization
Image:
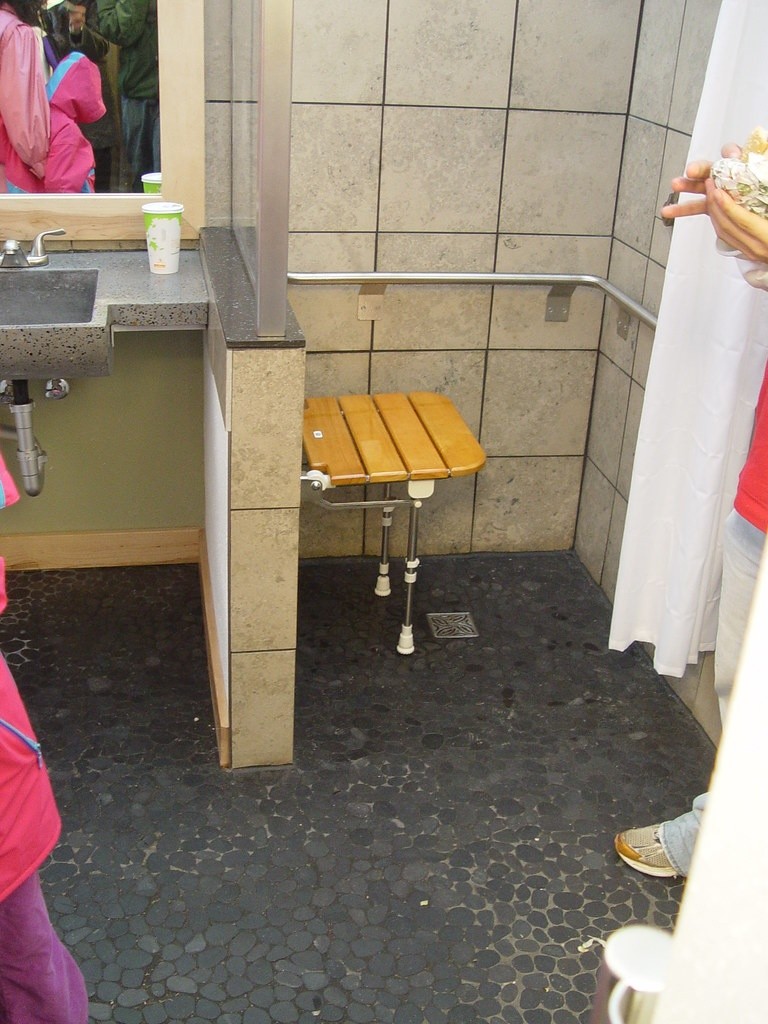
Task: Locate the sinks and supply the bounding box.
[0,255,112,380]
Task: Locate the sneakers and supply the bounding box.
[615,824,681,878]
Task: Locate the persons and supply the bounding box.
[615,133,768,881]
[0,0,161,193]
[0,452,89,1024]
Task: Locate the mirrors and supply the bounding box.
[0,0,206,241]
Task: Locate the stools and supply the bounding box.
[298,393,490,656]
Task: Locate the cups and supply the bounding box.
[142,202,184,275]
[141,172,162,193]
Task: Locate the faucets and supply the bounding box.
[0,228,67,269]
